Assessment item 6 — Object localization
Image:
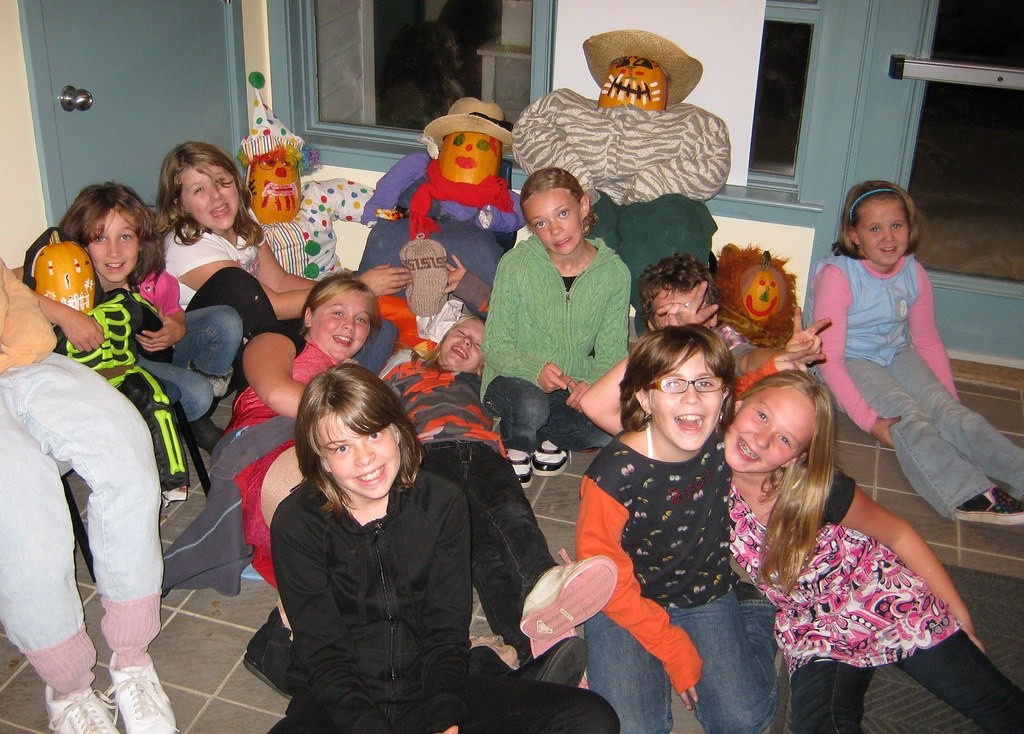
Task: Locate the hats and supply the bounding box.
[584,29,703,106]
[422,97,513,156]
[401,233,448,316]
[240,70,305,157]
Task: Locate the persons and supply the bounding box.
[158,141,412,356]
[230,71,408,281]
[513,29,731,339]
[481,168,633,489]
[578,322,827,734]
[383,318,619,687]
[0,257,177,734]
[23,229,192,506]
[268,365,620,734]
[581,256,832,435]
[60,181,243,454]
[582,280,1024,734]
[220,273,379,588]
[812,180,1024,523]
[355,96,526,374]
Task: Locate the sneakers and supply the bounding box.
[519,554,616,642]
[111,650,178,733]
[530,440,568,477]
[506,447,533,489]
[44,679,119,734]
[955,486,1024,525]
[528,627,589,691]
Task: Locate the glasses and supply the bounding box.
[644,377,726,394]
